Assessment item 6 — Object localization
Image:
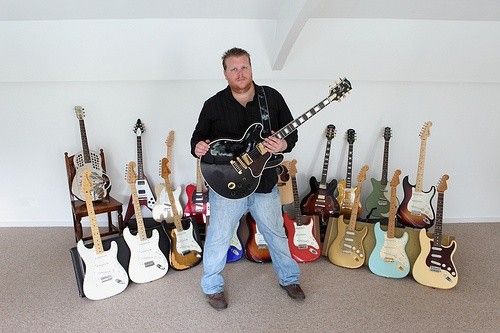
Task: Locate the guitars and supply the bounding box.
[225,219,244,263]
[282,159,322,263]
[275,160,295,207]
[158,157,203,272]
[70,105,112,204]
[300,123,341,217]
[183,159,210,224]
[395,121,437,228]
[123,119,157,224]
[121,161,168,284]
[364,127,400,218]
[369,168,410,280]
[152,130,185,224]
[334,129,363,219]
[327,165,370,269]
[76,168,129,300]
[411,174,460,290]
[199,77,354,200]
[244,211,273,264]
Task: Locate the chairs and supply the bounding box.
[64,149,123,244]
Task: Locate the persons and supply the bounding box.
[188,47,305,311]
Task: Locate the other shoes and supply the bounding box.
[208,291,228,311]
[280,283,306,300]
